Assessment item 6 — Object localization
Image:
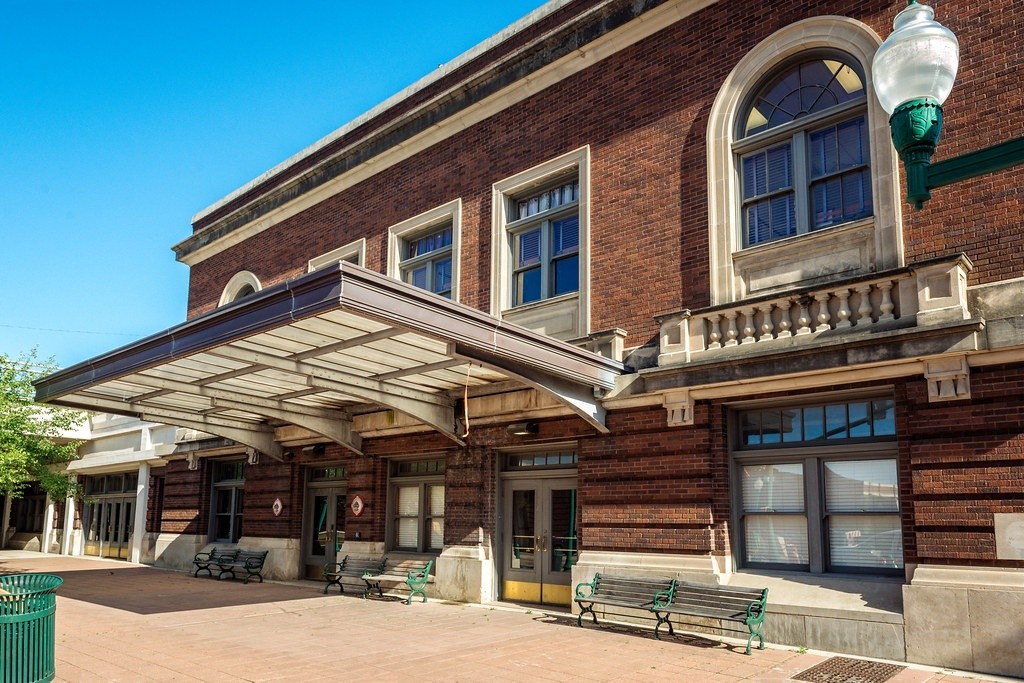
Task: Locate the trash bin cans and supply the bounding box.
[0,574,64,682]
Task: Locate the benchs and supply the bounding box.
[192,546,269,584]
[573,572,769,654]
[321,555,434,605]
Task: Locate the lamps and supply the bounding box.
[868,0,1024,209]
